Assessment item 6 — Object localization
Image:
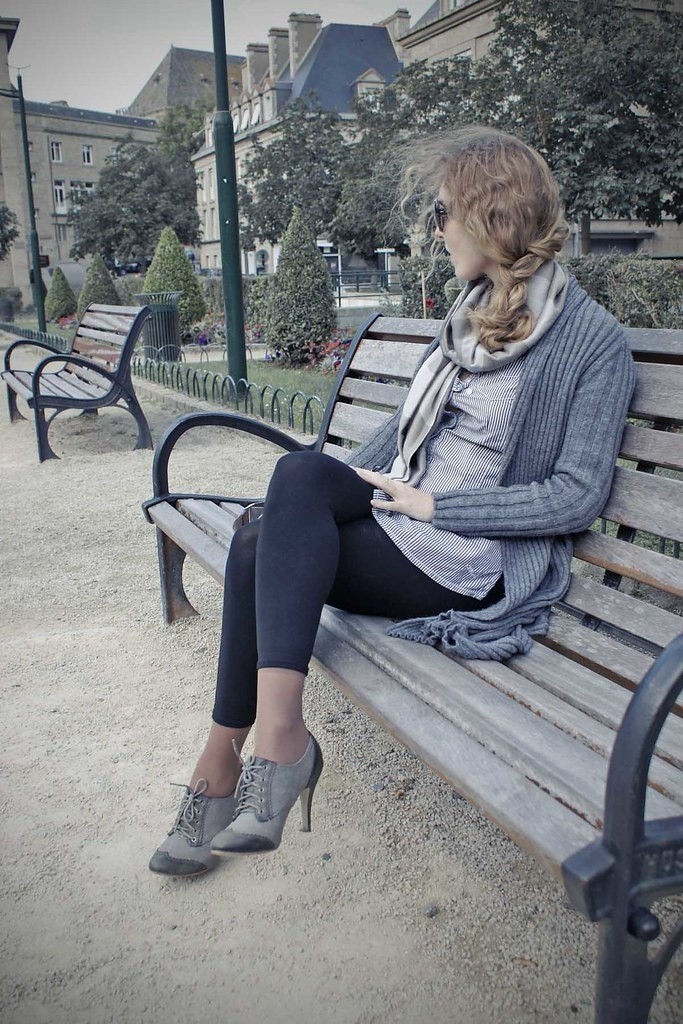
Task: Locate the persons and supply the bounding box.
[149,126,636,878]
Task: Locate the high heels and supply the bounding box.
[210,725,324,856]
[147,766,241,878]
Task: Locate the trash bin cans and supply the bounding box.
[133,291,183,362]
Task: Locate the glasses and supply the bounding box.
[434,198,451,231]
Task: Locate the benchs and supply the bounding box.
[1,302,155,463]
[140,311,683,1024]
